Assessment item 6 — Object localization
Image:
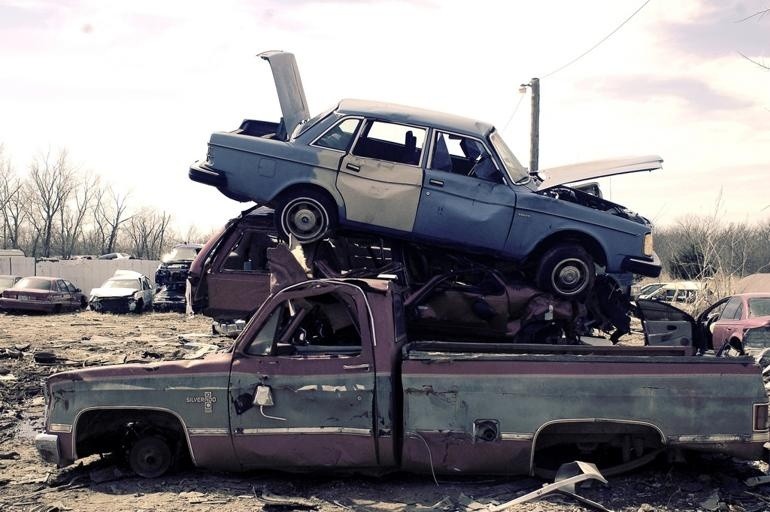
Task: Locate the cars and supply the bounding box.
[153,244,206,290]
[89,270,154,316]
[634,290,770,363]
[186,49,666,299]
[190,203,717,349]
[1,274,23,291]
[0,276,89,316]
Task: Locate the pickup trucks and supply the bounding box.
[31,275,769,484]
